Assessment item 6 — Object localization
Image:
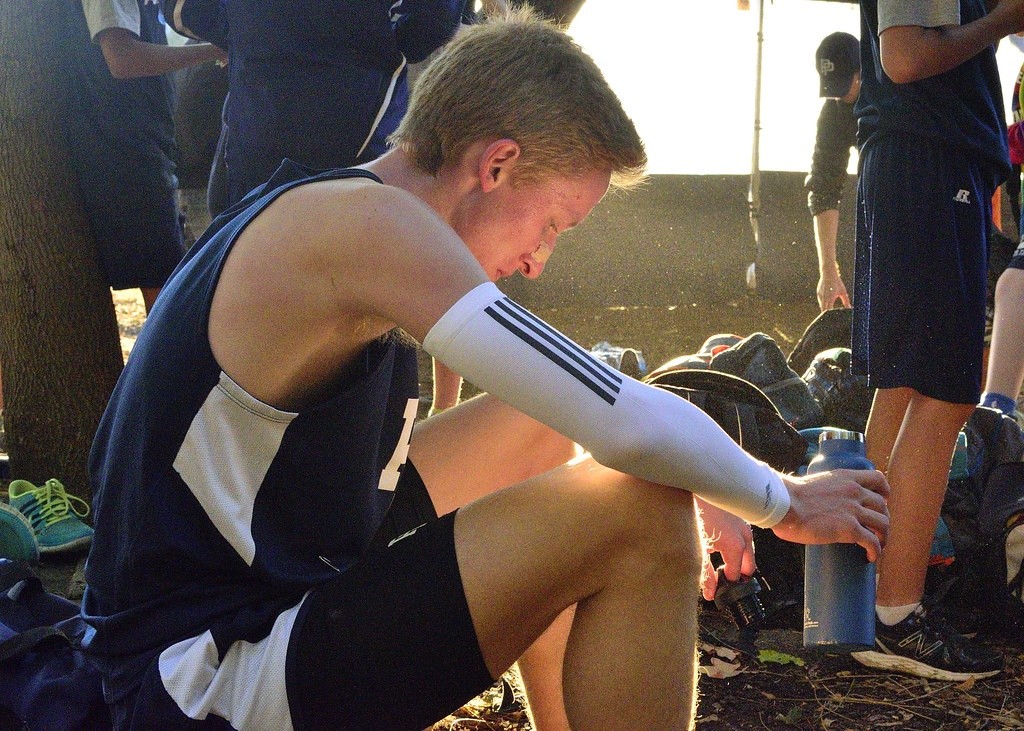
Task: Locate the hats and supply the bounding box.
[816,31,860,99]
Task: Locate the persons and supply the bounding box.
[61,0,597,320]
[847,0,1024,684]
[82,0,892,731]
[974,61,1024,429]
[805,28,869,314]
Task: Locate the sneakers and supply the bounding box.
[850,600,1006,681]
[9,479,94,553]
[0,502,39,564]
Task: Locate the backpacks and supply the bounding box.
[576,305,1024,637]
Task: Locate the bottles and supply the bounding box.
[803,431,877,654]
